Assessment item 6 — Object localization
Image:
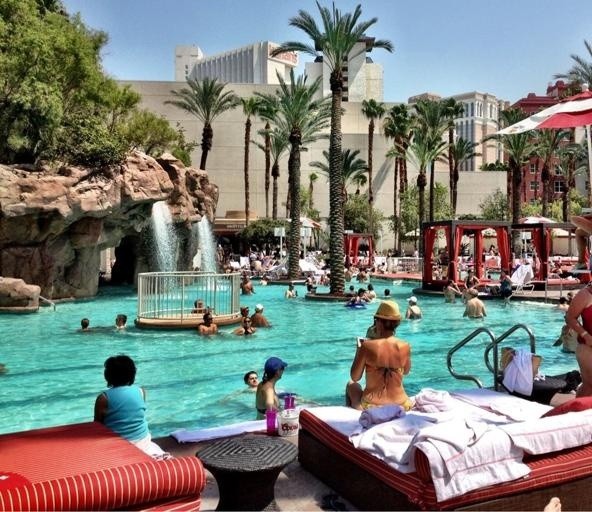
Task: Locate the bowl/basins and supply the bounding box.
[277,409,299,436]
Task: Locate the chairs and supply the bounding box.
[0,422,206,511]
[299,389,592,511]
[424,265,589,291]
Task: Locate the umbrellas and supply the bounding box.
[519,214,558,260]
[494,83,592,177]
[285,216,322,261]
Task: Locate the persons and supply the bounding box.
[220,371,260,405]
[115,314,127,332]
[346,302,413,412]
[198,313,217,337]
[191,299,212,315]
[94,356,173,461]
[565,281,592,397]
[81,318,90,331]
[255,357,288,416]
[212,228,592,352]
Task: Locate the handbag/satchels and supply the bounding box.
[500,346,541,379]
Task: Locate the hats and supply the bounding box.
[265,356,287,374]
[471,289,478,296]
[407,296,417,303]
[242,306,249,311]
[255,304,263,311]
[375,301,402,321]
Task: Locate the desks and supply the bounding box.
[192,435,297,512]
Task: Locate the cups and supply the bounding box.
[265,407,278,434]
[284,393,296,410]
